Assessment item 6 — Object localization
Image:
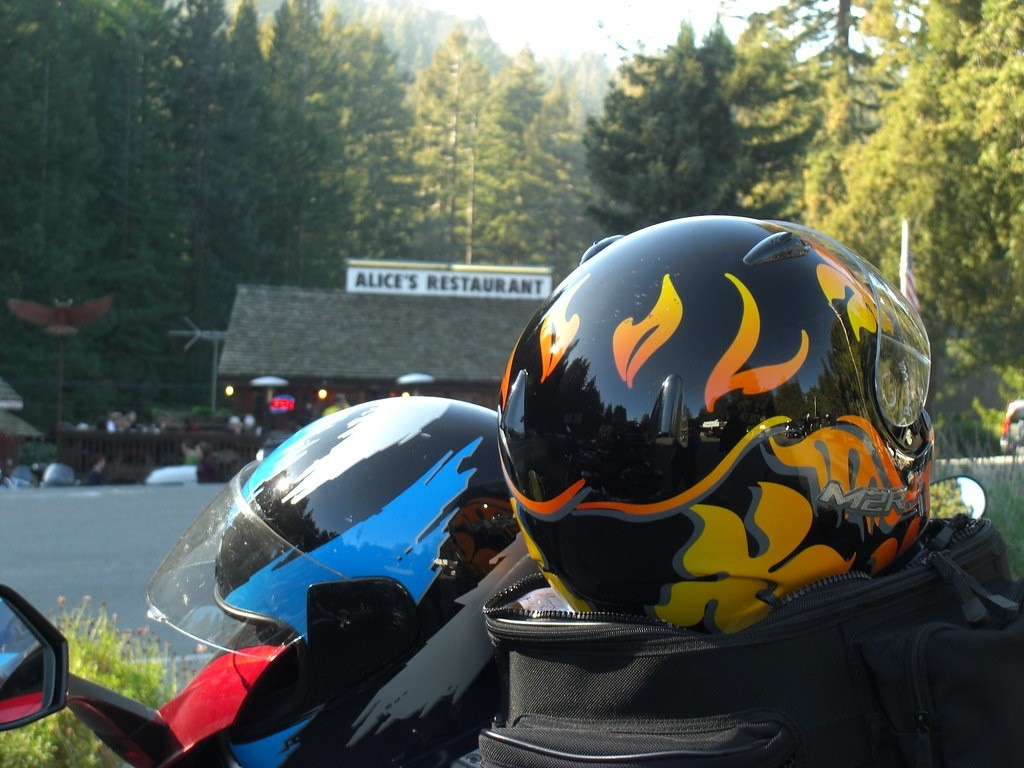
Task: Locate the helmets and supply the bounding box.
[497,215,933,636]
[214,395,545,768]
[1,604,41,683]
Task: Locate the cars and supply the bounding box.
[999,398,1024,456]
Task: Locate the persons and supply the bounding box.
[456,215,1024,768]
[141,396,545,768]
[97,409,137,432]
[227,414,262,436]
[182,442,210,465]
[323,393,350,417]
[87,454,106,486]
